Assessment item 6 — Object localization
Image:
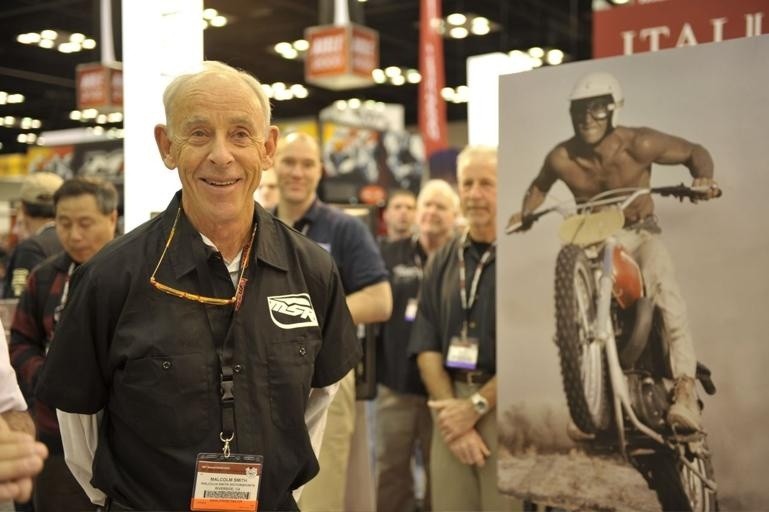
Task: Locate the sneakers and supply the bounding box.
[666,375,704,436]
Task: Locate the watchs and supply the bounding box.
[470,392,490,416]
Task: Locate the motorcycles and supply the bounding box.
[505,182,722,512]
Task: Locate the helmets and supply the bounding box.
[569,71,627,111]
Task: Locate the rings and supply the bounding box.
[711,184,718,189]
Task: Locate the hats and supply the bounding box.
[16,170,69,210]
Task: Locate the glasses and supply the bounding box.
[570,101,614,123]
[149,213,264,309]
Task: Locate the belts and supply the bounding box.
[450,371,492,385]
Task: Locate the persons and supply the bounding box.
[0,319,48,512]
[509,69,721,435]
[404,138,523,511]
[1,171,63,301]
[262,129,395,512]
[9,176,118,512]
[33,58,364,510]
[371,178,462,512]
[374,188,423,271]
[257,163,280,207]
